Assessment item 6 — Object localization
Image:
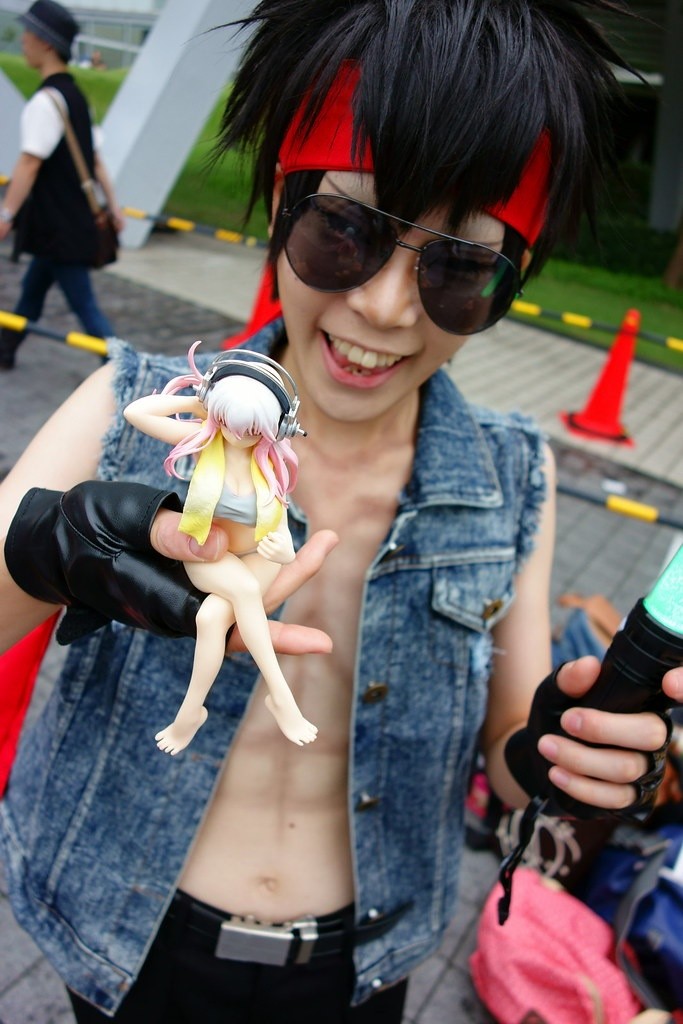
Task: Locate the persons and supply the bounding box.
[82,49,109,73]
[123,348,318,756]
[0,0,683,1024]
[0,0,123,371]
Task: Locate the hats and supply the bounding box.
[13,0,80,63]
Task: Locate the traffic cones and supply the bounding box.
[559,307,644,449]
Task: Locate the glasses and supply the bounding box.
[269,184,520,336]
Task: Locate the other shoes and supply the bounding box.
[1,338,17,370]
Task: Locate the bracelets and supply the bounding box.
[0,208,14,222]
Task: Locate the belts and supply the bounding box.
[164,894,350,968]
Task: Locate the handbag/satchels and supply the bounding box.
[76,204,120,271]
